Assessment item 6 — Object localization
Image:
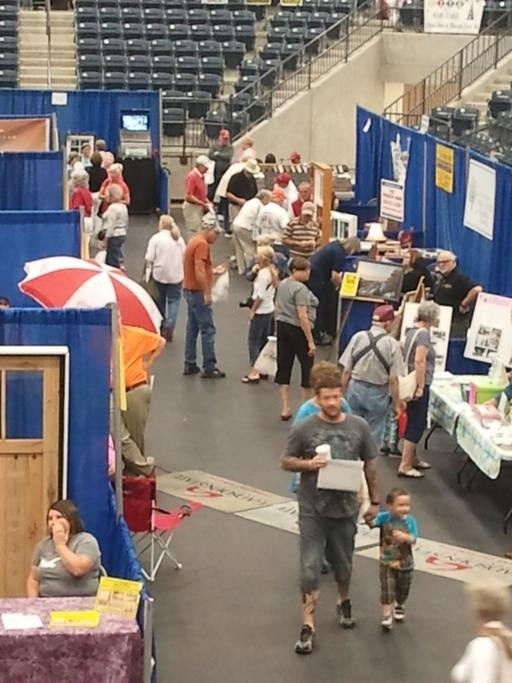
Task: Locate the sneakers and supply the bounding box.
[336,598,355,629]
[314,336,331,345]
[392,599,406,621]
[380,616,393,632]
[161,329,173,341]
[294,624,316,654]
[204,368,225,378]
[184,361,200,375]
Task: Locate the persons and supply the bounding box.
[363,486,418,632]
[65,140,132,268]
[450,571,512,683]
[339,304,440,478]
[402,249,483,341]
[279,379,381,655]
[25,498,101,598]
[145,130,362,421]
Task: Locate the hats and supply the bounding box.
[243,153,315,216]
[221,130,229,139]
[195,155,213,169]
[200,214,224,234]
[371,305,402,322]
[68,151,77,164]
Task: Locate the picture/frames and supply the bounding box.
[354,258,405,302]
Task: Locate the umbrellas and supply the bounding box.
[20,254,164,336]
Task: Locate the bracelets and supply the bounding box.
[371,501,380,506]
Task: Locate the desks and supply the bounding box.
[425,375,512,490]
[359,239,402,252]
[336,295,385,360]
[0,596,144,683]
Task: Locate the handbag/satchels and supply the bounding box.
[97,229,107,241]
[397,327,429,403]
[138,261,160,305]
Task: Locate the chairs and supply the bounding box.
[400,0,512,167]
[123,474,190,582]
[74,0,357,141]
[0,4,19,88]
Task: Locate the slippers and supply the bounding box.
[241,375,260,384]
[281,408,292,421]
[380,446,432,478]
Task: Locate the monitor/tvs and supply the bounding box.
[120,111,149,131]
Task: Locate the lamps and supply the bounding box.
[366,224,387,261]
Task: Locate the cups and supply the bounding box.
[315,444,332,462]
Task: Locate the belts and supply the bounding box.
[126,380,147,392]
